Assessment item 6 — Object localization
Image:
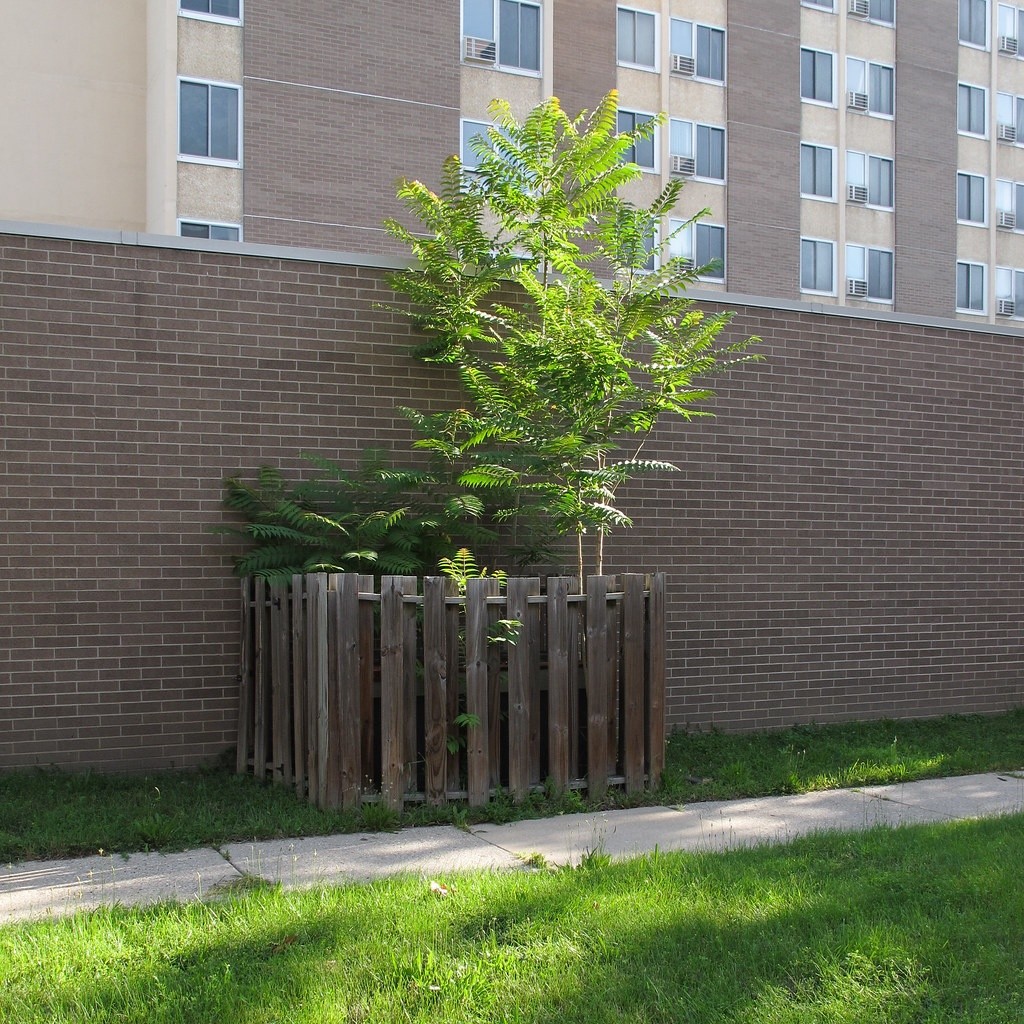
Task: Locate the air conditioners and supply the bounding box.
[464,36,496,65]
[996,212,1016,227]
[998,125,1017,140]
[670,255,695,276]
[995,299,1015,315]
[846,184,868,203]
[847,278,868,297]
[848,0,871,16]
[670,155,696,175]
[671,54,695,74]
[998,36,1018,54]
[847,91,869,109]
